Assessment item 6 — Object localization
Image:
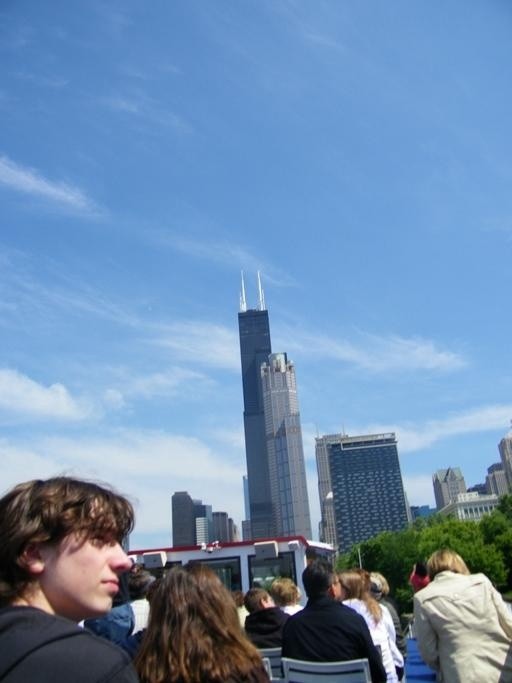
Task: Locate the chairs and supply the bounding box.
[258,647,372,683]
